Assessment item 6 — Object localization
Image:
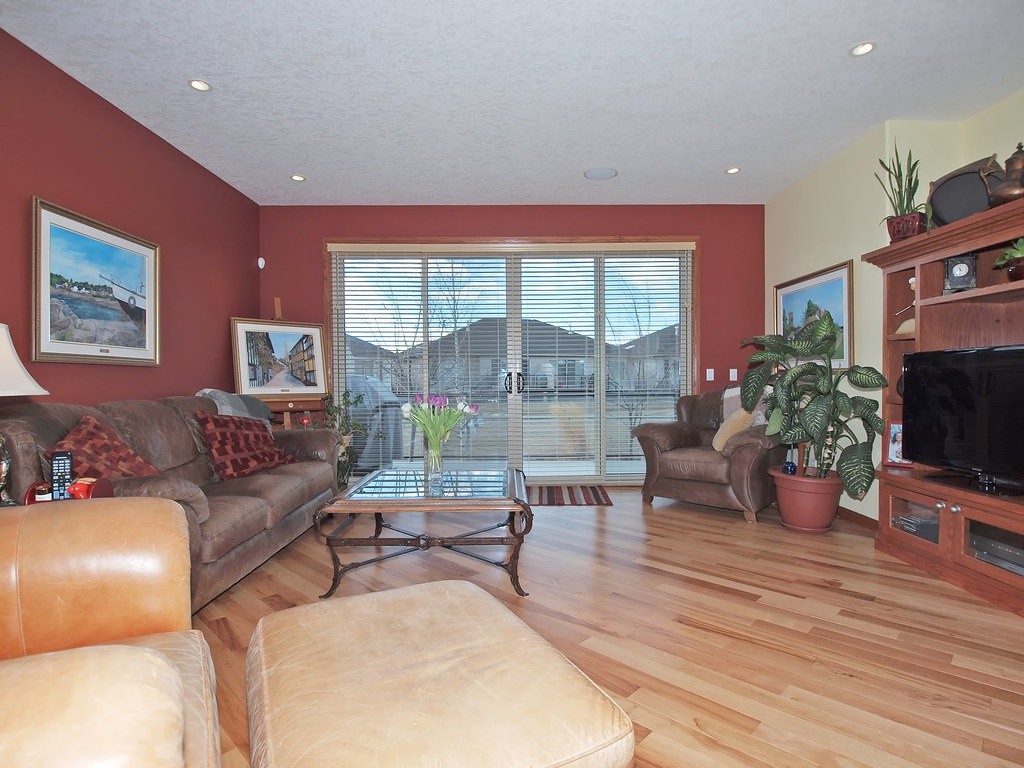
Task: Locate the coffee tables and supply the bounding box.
[313,469,533,599]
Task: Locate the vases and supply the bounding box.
[428,443,443,473]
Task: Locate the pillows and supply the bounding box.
[712,405,755,452]
[196,412,291,479]
[44,413,165,482]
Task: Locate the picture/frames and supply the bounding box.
[230,316,332,402]
[771,259,855,375]
[30,195,161,368]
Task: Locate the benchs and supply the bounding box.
[247,572,636,768]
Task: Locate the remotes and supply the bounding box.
[50,451,72,501]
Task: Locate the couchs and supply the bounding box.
[631,383,788,524]
[0,496,223,768]
[0,393,344,615]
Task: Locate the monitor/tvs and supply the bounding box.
[902,343,1024,497]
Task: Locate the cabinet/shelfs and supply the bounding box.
[862,195,1024,621]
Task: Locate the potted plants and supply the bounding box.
[873,135,934,241]
[739,311,888,534]
[995,238,1024,281]
[297,389,388,486]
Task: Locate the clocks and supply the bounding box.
[942,255,977,294]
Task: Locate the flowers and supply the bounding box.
[402,394,478,443]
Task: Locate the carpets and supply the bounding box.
[526,485,613,506]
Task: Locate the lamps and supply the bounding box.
[0,323,49,396]
